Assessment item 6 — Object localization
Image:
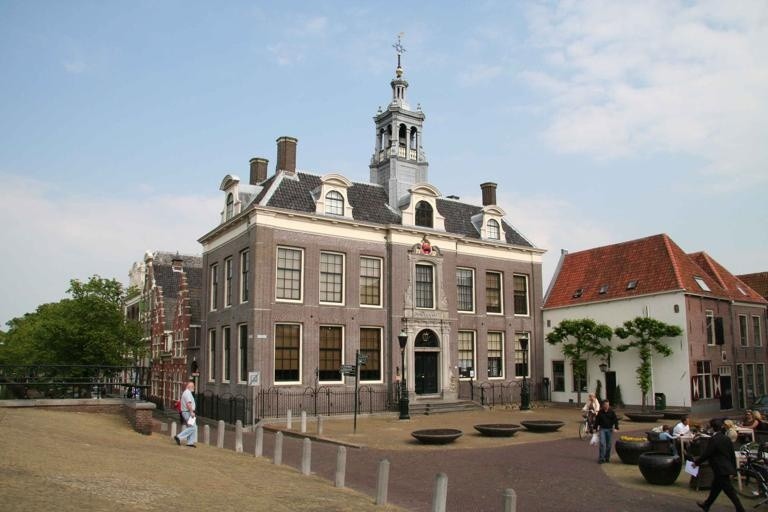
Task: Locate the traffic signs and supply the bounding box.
[192,371,201,377]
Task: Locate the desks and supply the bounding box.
[733,450,768,490]
[677,432,714,464]
[734,426,756,443]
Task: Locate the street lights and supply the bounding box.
[519,336,530,410]
[397,330,410,418]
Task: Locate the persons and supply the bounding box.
[173,381,198,447]
[581,393,601,434]
[723,418,739,443]
[592,399,620,463]
[420,234,432,255]
[659,424,681,456]
[689,418,747,511]
[737,409,763,434]
[175,397,187,426]
[673,416,690,438]
[742,408,755,426]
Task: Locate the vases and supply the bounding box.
[616,442,681,486]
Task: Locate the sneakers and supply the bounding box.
[174,435,196,448]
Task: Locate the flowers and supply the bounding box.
[621,436,648,442]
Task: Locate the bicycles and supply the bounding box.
[728,441,768,503]
[579,410,599,441]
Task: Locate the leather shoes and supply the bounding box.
[695,500,709,512]
